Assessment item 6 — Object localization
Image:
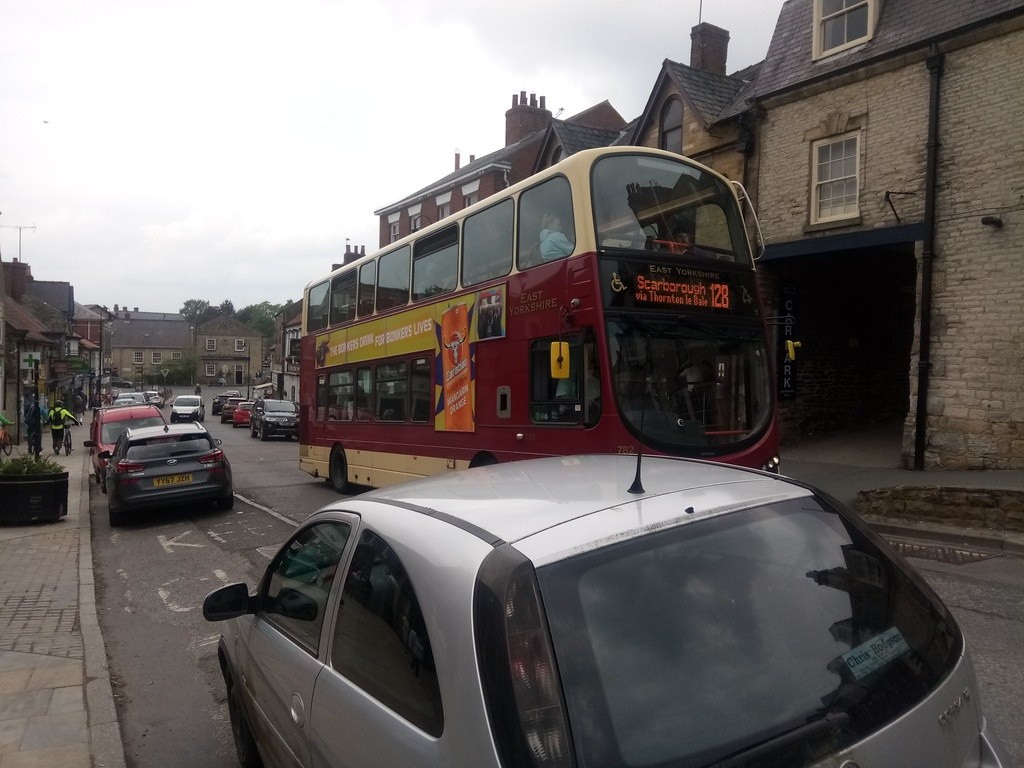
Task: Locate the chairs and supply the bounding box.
[319,240,540,328]
[322,394,430,421]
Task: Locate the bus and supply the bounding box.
[298,145,801,494]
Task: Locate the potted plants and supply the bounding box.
[0,453,69,527]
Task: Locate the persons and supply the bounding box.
[556,346,600,418]
[264,390,270,398]
[48,399,80,455]
[96,387,118,406]
[0,416,15,426]
[220,375,226,386]
[665,229,694,256]
[25,401,47,452]
[74,393,87,425]
[194,384,201,395]
[351,386,367,407]
[540,212,574,261]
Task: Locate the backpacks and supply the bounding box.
[74,394,85,407]
[52,407,65,426]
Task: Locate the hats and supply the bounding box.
[55,400,65,407]
[196,383,200,385]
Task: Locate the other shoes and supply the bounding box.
[55,446,59,455]
[39,448,44,452]
[80,421,83,426]
[27,449,32,454]
[54,450,57,455]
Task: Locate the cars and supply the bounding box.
[111,376,133,389]
[205,448,1014,768]
[82,390,233,526]
[211,390,301,440]
[169,396,206,423]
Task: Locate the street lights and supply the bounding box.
[96,306,114,407]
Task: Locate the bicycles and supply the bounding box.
[61,421,82,456]
[1,423,18,457]
